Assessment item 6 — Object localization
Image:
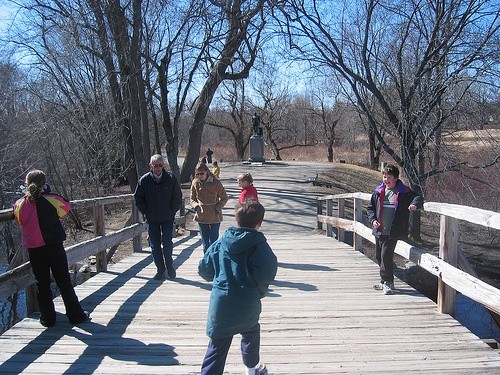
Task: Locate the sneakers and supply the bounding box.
[383,281,393,294]
[373,283,383,289]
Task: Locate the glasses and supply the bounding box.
[196,173,205,175]
[153,164,163,167]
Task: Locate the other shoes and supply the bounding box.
[168,271,176,278]
[70,311,89,323]
[154,272,163,279]
[39,314,55,327]
[255,364,268,375]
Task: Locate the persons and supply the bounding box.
[133,154,182,280]
[206,148,214,163]
[367,165,424,295]
[252,112,261,136]
[210,161,220,178]
[198,199,277,375]
[202,156,206,163]
[190,163,228,254]
[238,172,259,203]
[13,170,90,327]
